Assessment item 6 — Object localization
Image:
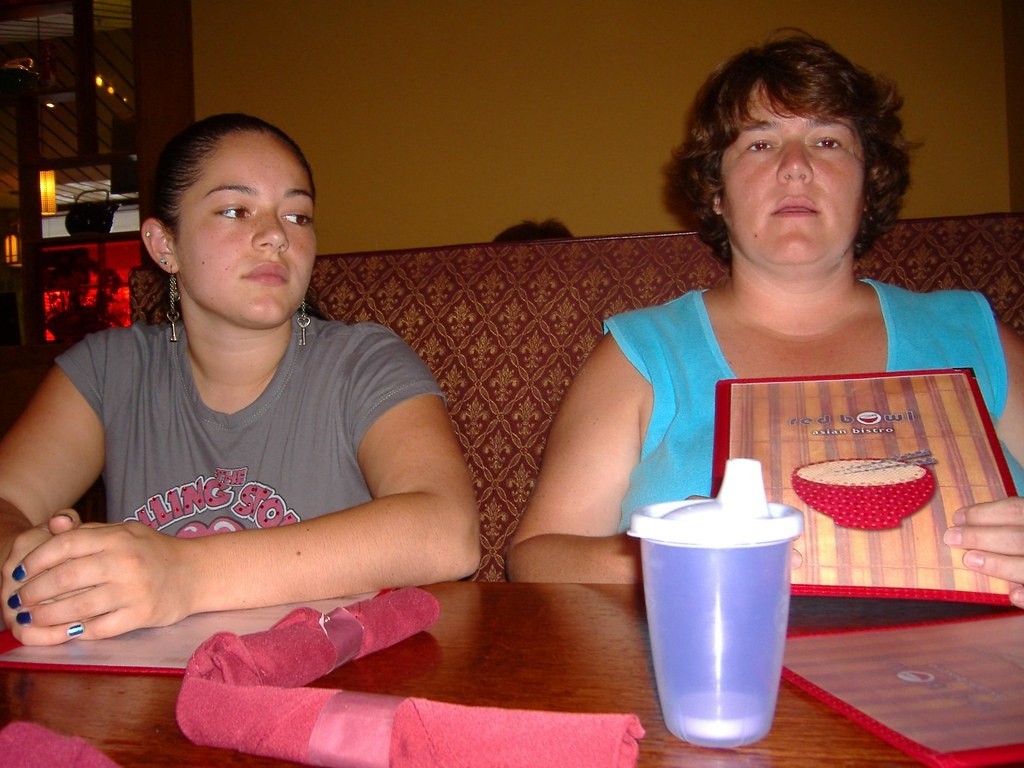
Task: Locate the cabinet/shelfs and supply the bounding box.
[0,0,195,364]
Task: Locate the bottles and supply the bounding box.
[626,458,804,747]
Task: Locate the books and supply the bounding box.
[781,608,1024,768]
[706,368,1024,607]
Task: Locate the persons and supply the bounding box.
[0,113,483,648]
[506,27,1024,616]
[494,218,572,239]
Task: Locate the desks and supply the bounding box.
[0,581,1024,768]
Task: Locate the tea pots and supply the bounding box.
[64,187,122,236]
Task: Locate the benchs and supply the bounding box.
[127,209,1022,581]
